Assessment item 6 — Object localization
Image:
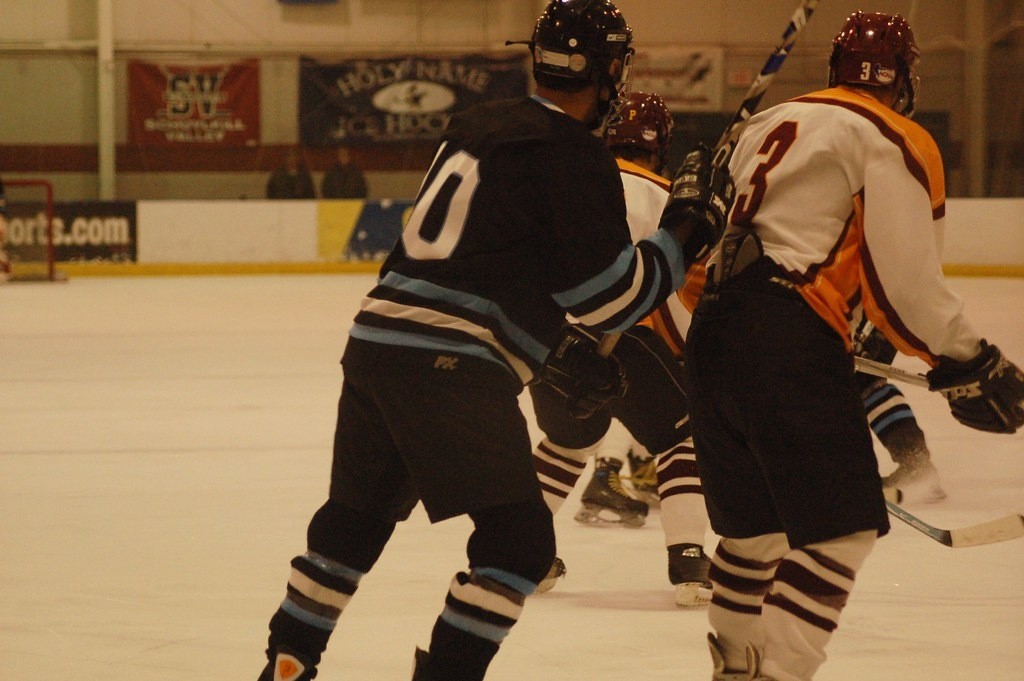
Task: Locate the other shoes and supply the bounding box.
[466,557,566,594]
[666,543,713,609]
[881,464,948,505]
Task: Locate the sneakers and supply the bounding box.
[573,458,649,528]
[619,448,661,508]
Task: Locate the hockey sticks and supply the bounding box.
[593,0,821,359]
[853,356,932,389]
[883,499,1023,550]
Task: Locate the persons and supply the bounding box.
[259,0,1023,681]
[322,147,370,199]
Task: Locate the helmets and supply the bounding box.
[601,91,676,152]
[829,9,919,86]
[506,0,636,85]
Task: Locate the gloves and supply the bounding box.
[541,329,628,419]
[658,141,736,247]
[927,338,1024,433]
